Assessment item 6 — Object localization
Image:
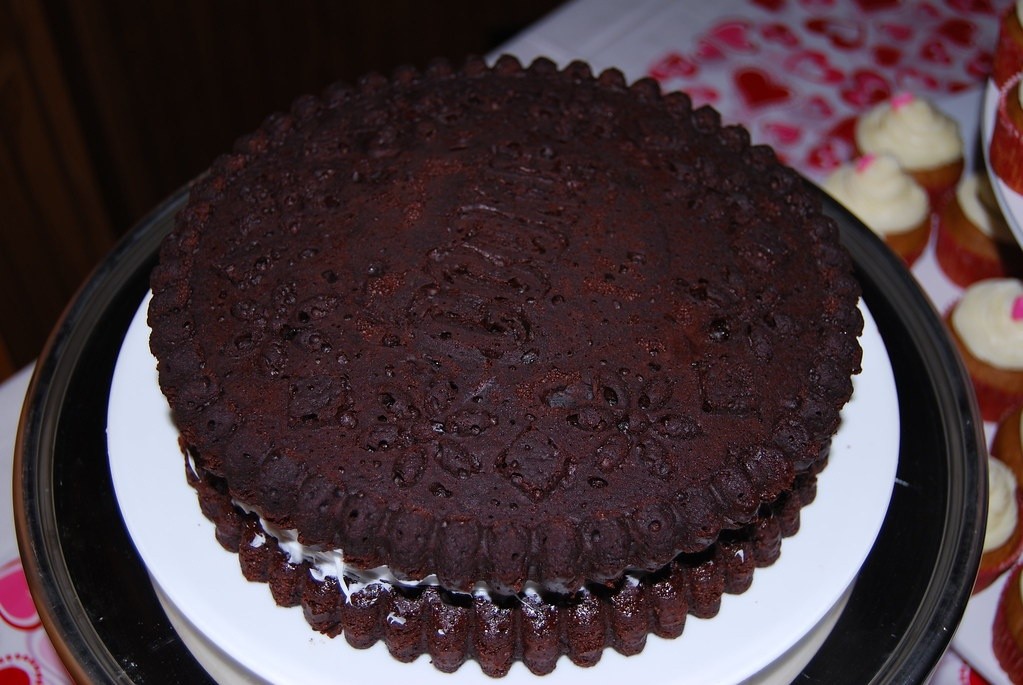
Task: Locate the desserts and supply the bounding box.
[815,85,1023,685]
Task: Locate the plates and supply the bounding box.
[108,287,898,685]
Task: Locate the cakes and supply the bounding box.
[147,56,865,677]
